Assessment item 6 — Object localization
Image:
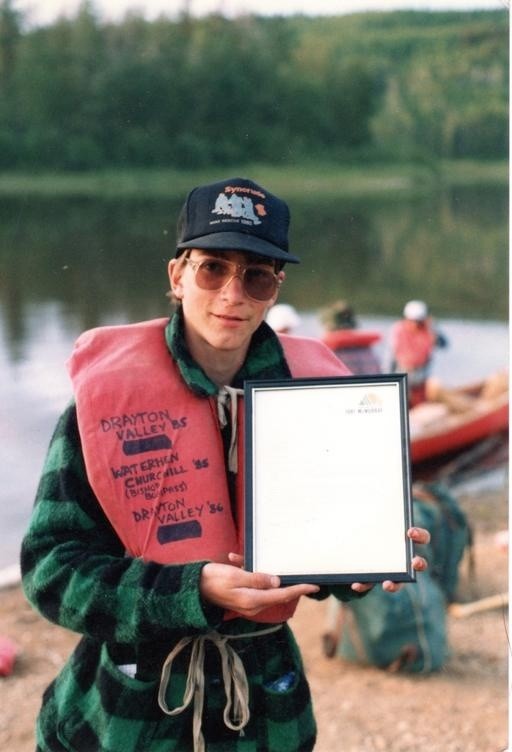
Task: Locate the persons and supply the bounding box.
[14,174,438,752]
[391,296,449,409]
[314,293,386,378]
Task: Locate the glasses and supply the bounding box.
[182,256,280,305]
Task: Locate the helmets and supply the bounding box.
[264,302,303,334]
[402,298,430,322]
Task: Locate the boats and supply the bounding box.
[408,370,509,486]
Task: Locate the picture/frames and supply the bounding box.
[243,372,419,588]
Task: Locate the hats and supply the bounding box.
[173,177,302,266]
